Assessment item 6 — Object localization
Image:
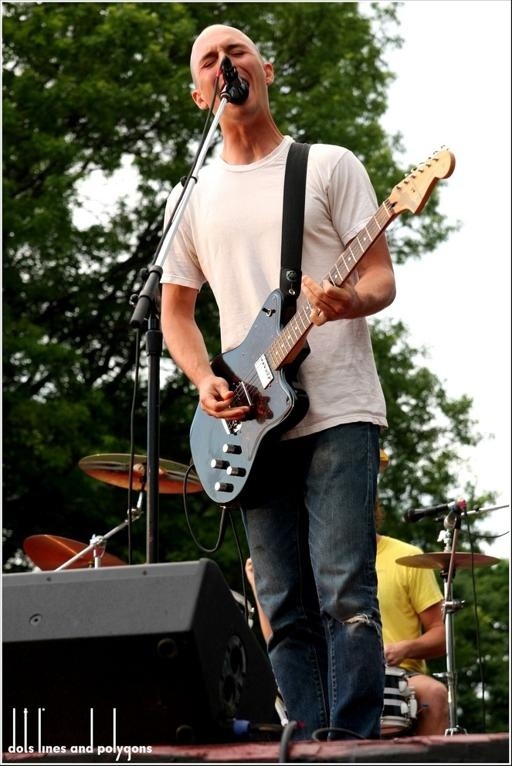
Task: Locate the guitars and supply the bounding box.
[189,145,455,507]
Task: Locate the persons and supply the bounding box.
[244,448,449,737]
[159,24,395,745]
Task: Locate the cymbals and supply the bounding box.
[394,552,502,570]
[79,453,204,495]
[22,535,124,570]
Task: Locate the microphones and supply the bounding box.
[220,54,249,106]
[403,497,463,524]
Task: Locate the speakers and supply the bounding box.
[3,559,279,752]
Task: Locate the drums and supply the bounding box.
[376,661,418,735]
